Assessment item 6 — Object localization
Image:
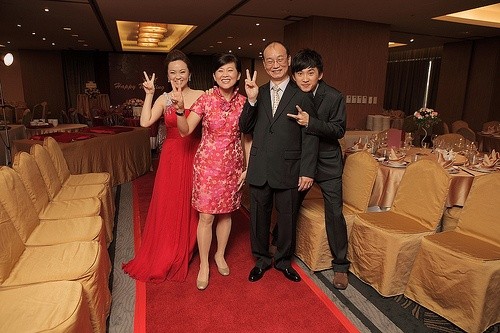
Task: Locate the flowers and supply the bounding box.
[126,98,144,107]
[413,107,442,129]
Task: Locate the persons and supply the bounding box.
[271,48,351,289]
[171,54,253,290]
[238,40,320,282]
[121,48,217,285]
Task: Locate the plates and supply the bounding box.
[382,162,407,168]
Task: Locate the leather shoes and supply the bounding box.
[248,265,263,281]
[274,264,301,282]
[334,272,348,288]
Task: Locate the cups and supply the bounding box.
[29,119,58,127]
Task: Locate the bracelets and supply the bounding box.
[176,110,185,116]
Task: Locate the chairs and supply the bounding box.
[293,109,500,333]
[0,101,125,333]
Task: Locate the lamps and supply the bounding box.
[0,44,14,67]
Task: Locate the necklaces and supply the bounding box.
[172,89,190,97]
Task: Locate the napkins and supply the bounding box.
[389,148,406,166]
[438,152,453,169]
[482,148,500,167]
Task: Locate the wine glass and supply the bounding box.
[353,132,479,169]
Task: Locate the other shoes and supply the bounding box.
[197,263,211,290]
[214,253,230,276]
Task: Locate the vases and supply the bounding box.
[420,124,433,137]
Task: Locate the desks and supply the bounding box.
[76,94,111,118]
[11,126,152,186]
[477,131,500,153]
[130,106,143,117]
[338,128,382,153]
[343,146,500,214]
